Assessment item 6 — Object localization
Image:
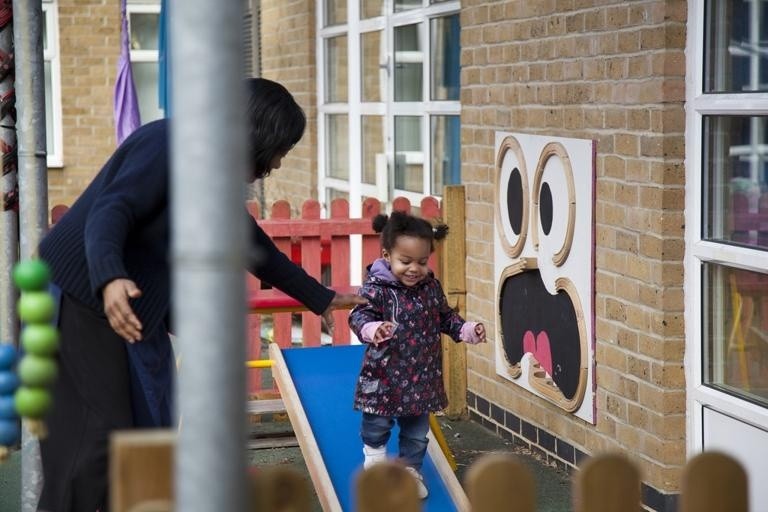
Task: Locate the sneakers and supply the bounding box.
[362,444,386,470]
[403,466,428,500]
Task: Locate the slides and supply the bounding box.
[266,341,469,512]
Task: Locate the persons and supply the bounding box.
[348,212,487,500]
[34,73,379,512]
